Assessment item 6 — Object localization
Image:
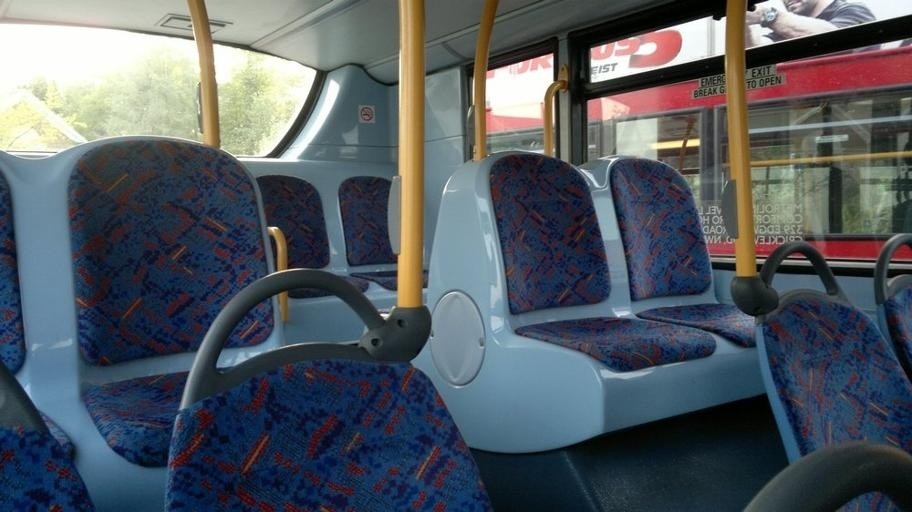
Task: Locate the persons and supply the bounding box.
[746,0,877,48]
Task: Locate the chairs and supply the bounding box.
[242,155,432,347]
[166,267,490,509]
[358,151,764,451]
[0,137,286,511]
[743,233,912,509]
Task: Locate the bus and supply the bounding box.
[472,46,912,262]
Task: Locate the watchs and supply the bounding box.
[760,6,780,27]
